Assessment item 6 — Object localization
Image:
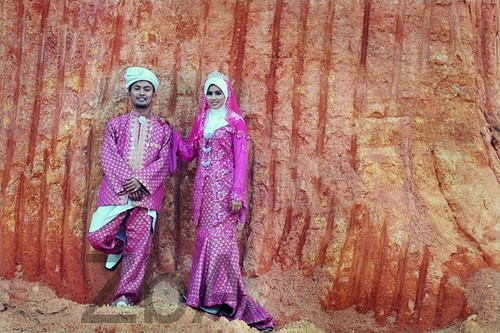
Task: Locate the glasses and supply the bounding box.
[206,90,222,95]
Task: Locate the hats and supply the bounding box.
[124,67,159,93]
[203,77,228,100]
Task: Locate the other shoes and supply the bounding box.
[182,293,224,317]
[105,252,123,270]
[114,300,129,306]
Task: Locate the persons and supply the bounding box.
[88,66,173,309]
[164,71,275,333]
